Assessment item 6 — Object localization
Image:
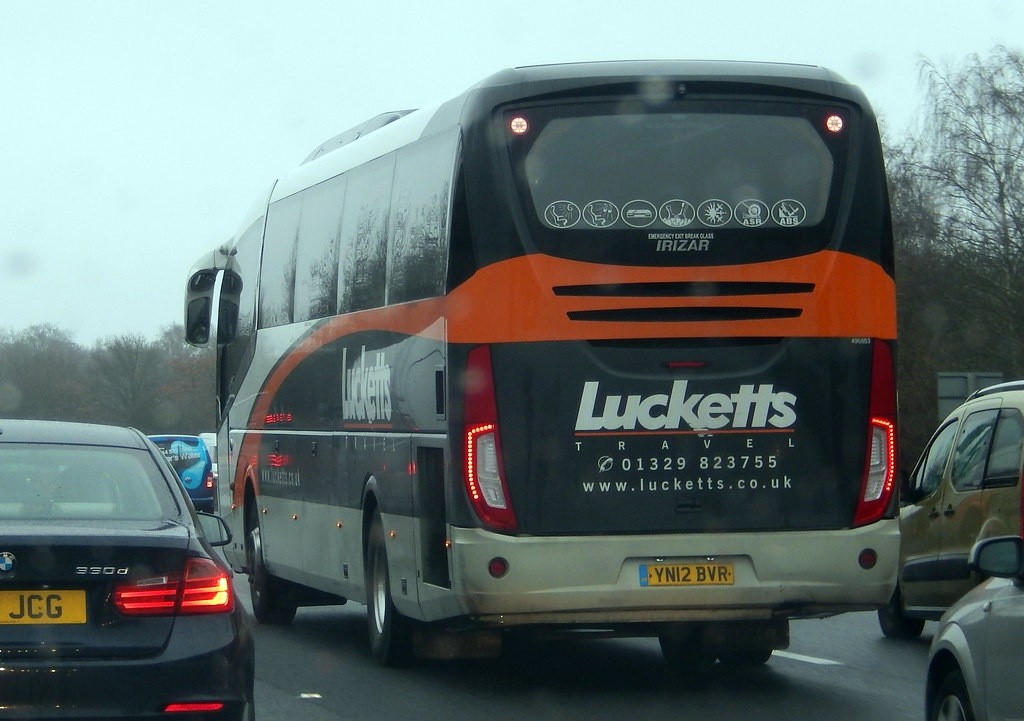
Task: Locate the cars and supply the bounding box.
[258,423,342,506]
[922,533,1023,720]
[170,433,218,489]
[308,411,420,515]
[144,433,215,515]
[1,418,257,721]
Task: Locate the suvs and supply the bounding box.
[877,379,1023,643]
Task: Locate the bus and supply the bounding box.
[184,60,903,670]
